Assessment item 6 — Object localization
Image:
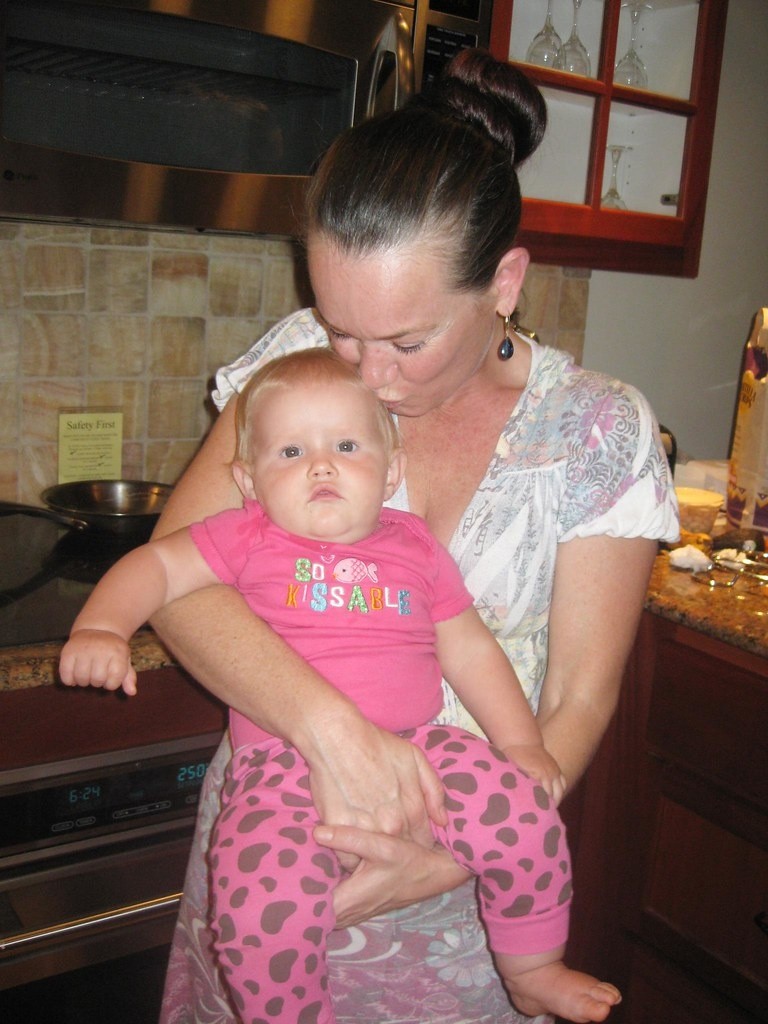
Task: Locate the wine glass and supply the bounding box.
[552,0,591,77]
[614,1,655,89]
[525,0,566,71]
[601,145,633,211]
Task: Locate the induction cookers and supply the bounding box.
[0,514,154,646]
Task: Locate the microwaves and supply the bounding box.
[0,0,492,241]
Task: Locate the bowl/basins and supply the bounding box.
[675,486,724,534]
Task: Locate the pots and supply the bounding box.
[1,479,175,539]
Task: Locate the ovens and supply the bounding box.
[0,733,222,1024]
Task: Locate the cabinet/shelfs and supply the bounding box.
[487,0,730,280]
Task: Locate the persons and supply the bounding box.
[142,51,678,1024]
[58,348,623,1024]
